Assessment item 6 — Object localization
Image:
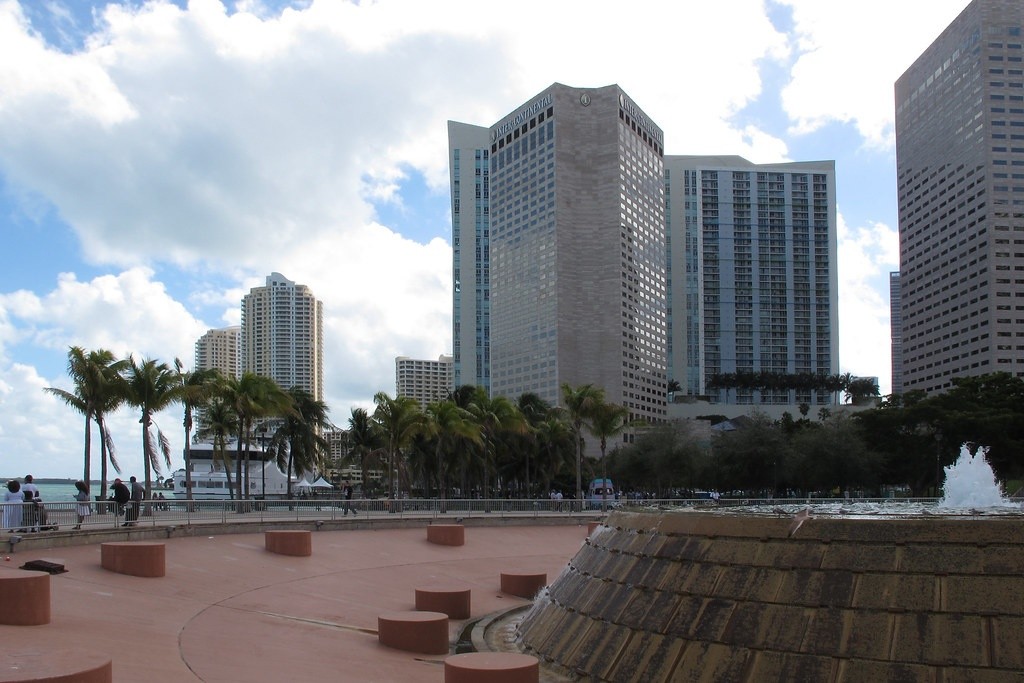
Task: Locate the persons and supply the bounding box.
[2,481,25,533]
[569,490,586,509]
[506,491,512,512]
[713,490,720,502]
[844,488,849,498]
[107,479,130,516]
[342,481,357,517]
[22,475,39,533]
[615,492,618,499]
[121,476,146,526]
[645,490,655,498]
[152,492,165,509]
[551,490,563,512]
[72,482,90,529]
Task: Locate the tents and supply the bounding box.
[294,477,333,494]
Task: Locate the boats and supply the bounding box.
[173,433,301,506]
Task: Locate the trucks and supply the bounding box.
[585,479,615,509]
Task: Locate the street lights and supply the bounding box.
[258,428,269,499]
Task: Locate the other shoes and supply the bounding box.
[72,526,81,529]
[118,510,125,516]
[353,513,358,516]
[53,523,58,530]
[121,523,127,526]
[8,530,36,533]
[341,514,347,517]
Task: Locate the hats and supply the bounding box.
[25,475,33,482]
[114,478,123,484]
